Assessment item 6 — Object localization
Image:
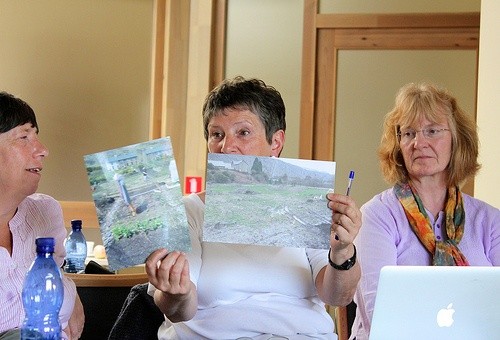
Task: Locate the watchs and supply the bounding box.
[328,244,357,271]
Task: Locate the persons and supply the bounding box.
[347,83,500,340]
[0,92,85,340]
[144,74,362,340]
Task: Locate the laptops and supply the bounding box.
[368,266,499,340]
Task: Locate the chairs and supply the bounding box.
[65,273,149,340]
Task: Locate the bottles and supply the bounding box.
[63,219,87,273]
[20,237,66,340]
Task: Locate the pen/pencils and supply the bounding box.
[334,171,355,240]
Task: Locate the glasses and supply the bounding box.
[398,127,449,141]
